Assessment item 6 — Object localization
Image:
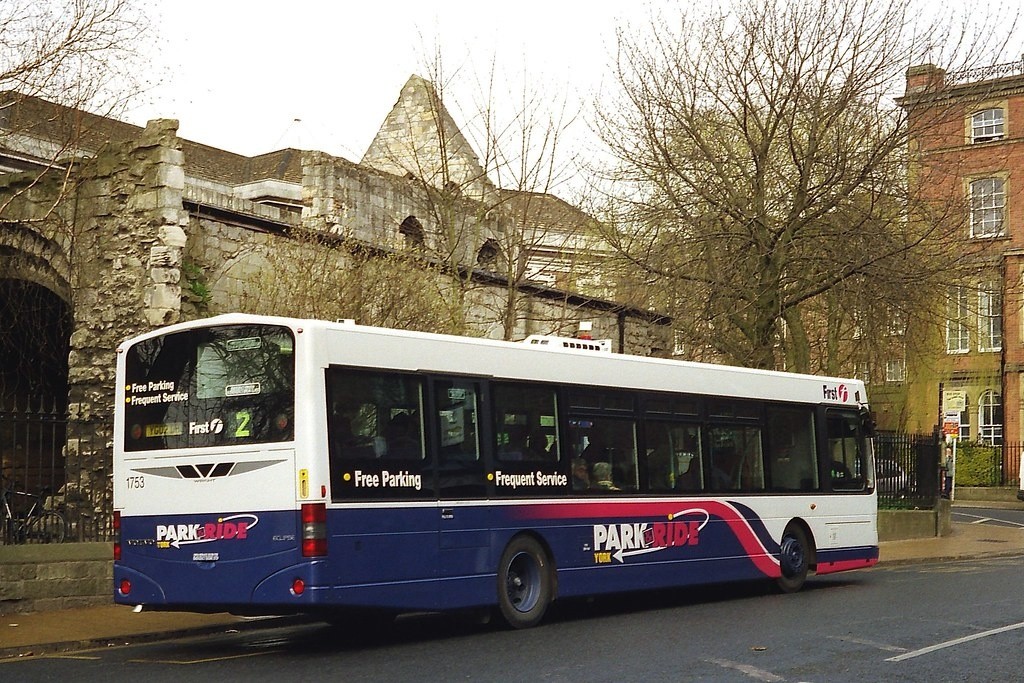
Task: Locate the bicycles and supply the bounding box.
[0,474,66,544]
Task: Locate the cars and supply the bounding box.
[1016,450,1024,501]
[855,459,917,493]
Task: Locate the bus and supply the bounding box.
[113,311,880,629]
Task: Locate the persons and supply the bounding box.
[326,409,744,490]
[942,455,953,499]
